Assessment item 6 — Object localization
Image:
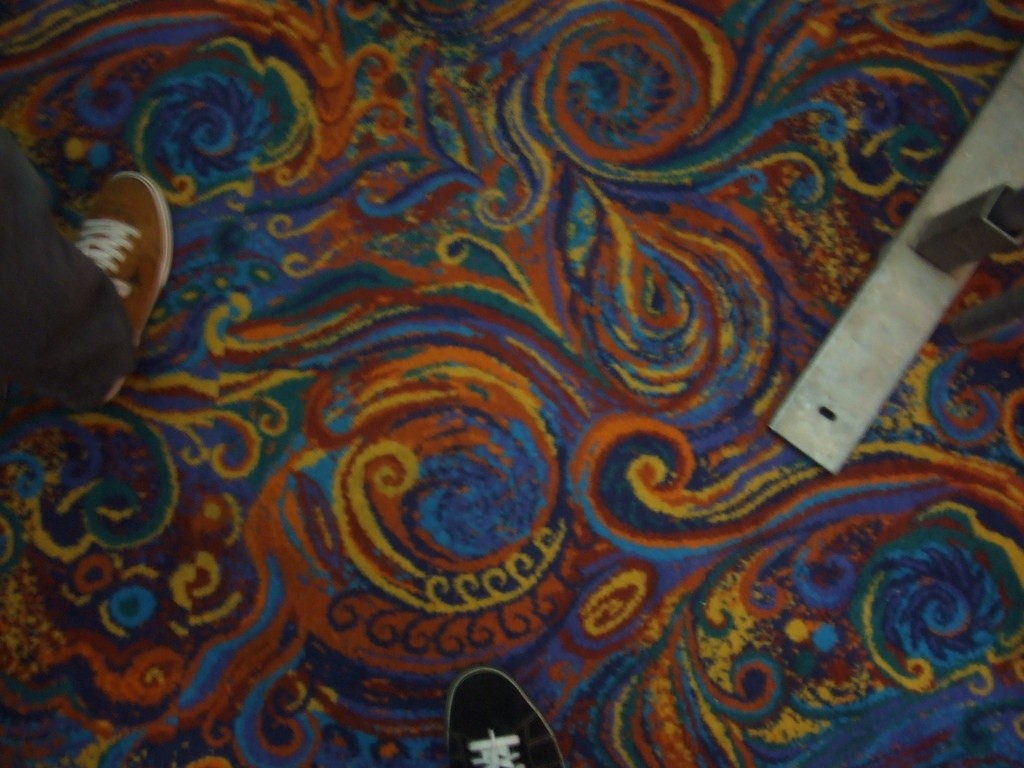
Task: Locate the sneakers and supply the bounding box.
[71,171,173,406]
[443,666,564,768]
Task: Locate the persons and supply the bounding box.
[445,665,565,767]
[1,171,174,406]
